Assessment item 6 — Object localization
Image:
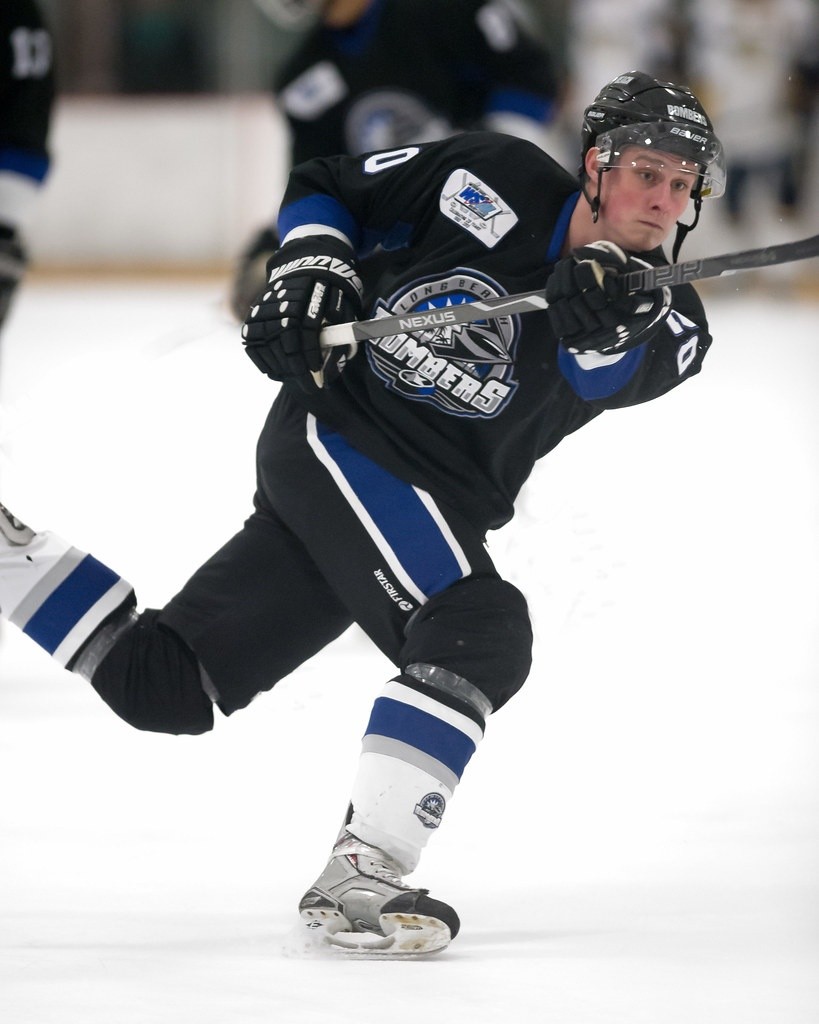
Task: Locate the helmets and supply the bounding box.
[579,70,721,171]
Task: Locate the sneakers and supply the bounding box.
[298,798,460,959]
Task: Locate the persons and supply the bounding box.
[0,69,729,959]
[0,0,61,328]
[235,1,568,315]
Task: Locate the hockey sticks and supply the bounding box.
[317,231,819,349]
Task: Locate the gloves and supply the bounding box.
[242,234,366,396]
[548,239,674,351]
